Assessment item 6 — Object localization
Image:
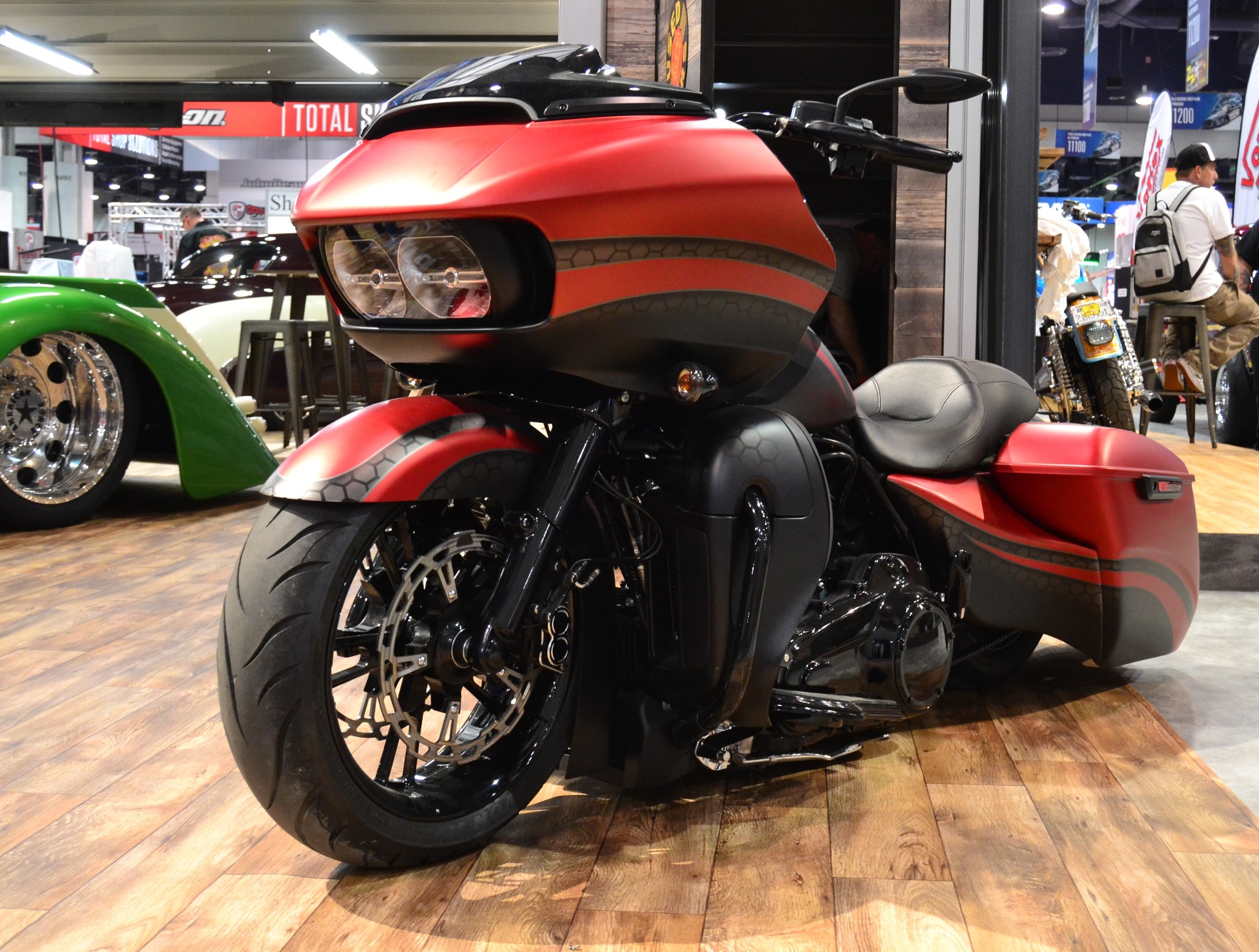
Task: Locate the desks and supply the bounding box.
[250,272,373,423]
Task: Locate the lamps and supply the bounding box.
[0,24,93,77]
[310,23,379,78]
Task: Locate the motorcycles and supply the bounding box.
[1035,198,1167,433]
[212,41,1206,875]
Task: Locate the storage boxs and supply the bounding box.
[991,421,1201,668]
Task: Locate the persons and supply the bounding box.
[1235,218,1259,343]
[1139,142,1259,394]
[173,207,242,276]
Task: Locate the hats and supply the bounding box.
[1174,143,1228,171]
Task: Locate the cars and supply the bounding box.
[119,232,379,427]
[0,272,279,534]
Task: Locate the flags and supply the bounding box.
[1132,90,1173,269]
[1231,46,1259,242]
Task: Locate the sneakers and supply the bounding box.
[1164,355,1185,391]
[1176,357,1205,393]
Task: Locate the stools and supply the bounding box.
[1135,303,1218,449]
[233,320,335,451]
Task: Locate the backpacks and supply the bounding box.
[1131,184,1215,301]
